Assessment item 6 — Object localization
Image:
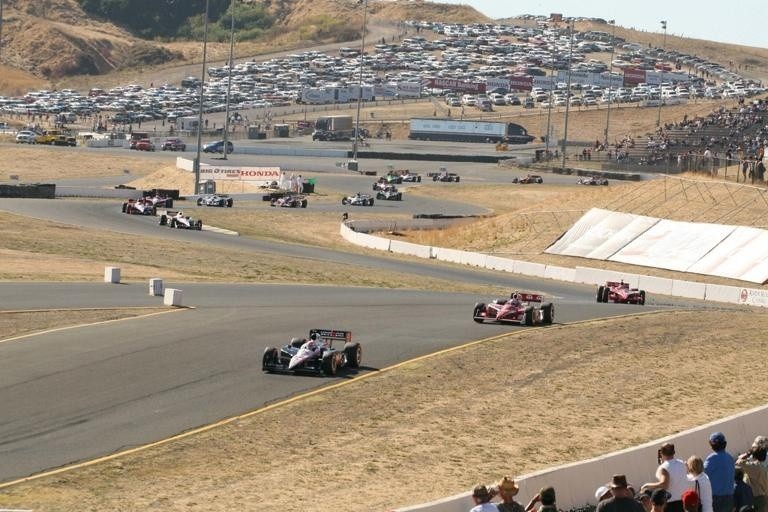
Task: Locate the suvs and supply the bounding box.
[128,132,151,149]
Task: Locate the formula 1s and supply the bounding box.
[342,169,460,207]
[473,287,555,327]
[578,176,608,186]
[196,193,233,208]
[270,194,308,209]
[262,328,363,377]
[159,210,202,230]
[142,192,173,208]
[512,174,543,184]
[596,279,645,305]
[122,198,156,216]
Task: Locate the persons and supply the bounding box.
[469,431,768,512]
[280,171,304,194]
[580,96,768,180]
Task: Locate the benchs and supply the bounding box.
[567,108,767,172]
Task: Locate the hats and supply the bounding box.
[681,490,699,506]
[710,432,725,445]
[595,474,635,501]
[752,435,768,448]
[651,489,672,502]
[638,488,651,499]
[473,475,519,503]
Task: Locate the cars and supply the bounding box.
[202,140,233,154]
[135,139,155,152]
[0,15,768,146]
[161,138,186,152]
